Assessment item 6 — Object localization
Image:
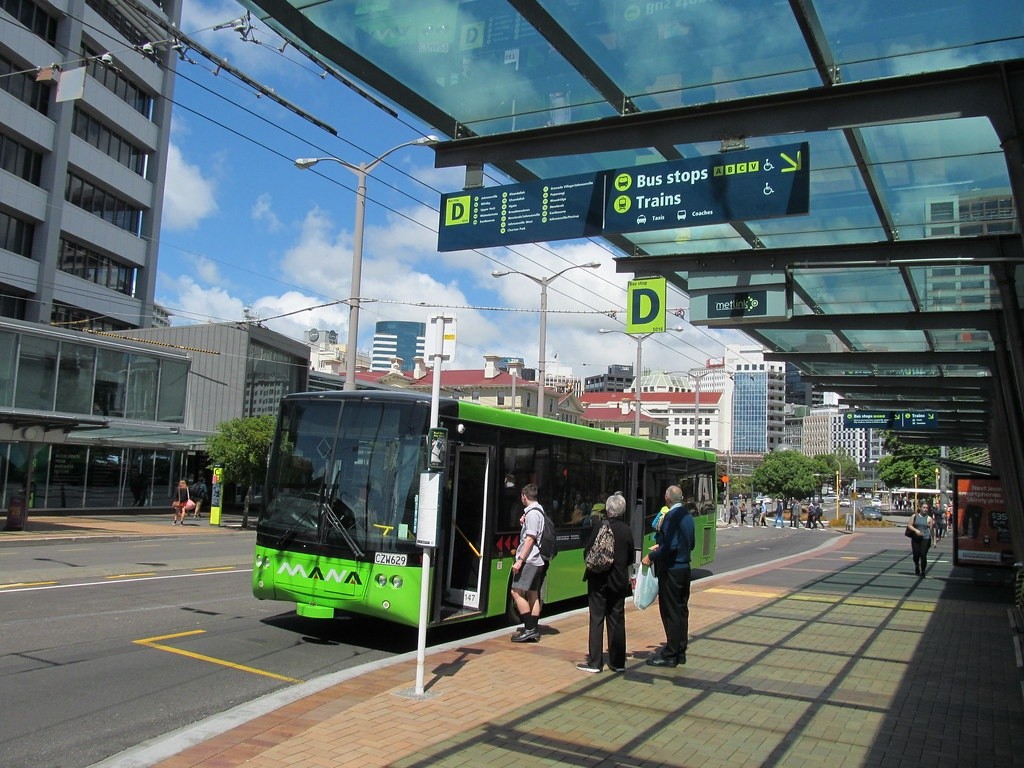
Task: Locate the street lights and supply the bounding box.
[726,437,746,524]
[599,326,683,438]
[663,371,733,449]
[295,135,439,390]
[491,262,601,419]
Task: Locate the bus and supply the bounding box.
[253,391,717,630]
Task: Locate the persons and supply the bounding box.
[194,477,209,519]
[129,471,150,507]
[549,491,610,526]
[893,496,953,578]
[511,484,551,642]
[722,493,825,529]
[172,480,191,527]
[576,490,634,672]
[642,485,695,667]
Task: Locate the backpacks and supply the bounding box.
[525,508,557,560]
[584,520,615,573]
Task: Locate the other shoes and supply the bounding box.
[646,655,678,668]
[576,663,600,673]
[607,662,625,671]
[677,654,686,663]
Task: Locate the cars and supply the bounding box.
[864,493,881,507]
[821,494,838,502]
[839,498,851,506]
[858,507,882,521]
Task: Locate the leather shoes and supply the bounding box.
[511,626,539,642]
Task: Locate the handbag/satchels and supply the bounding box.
[630,560,659,610]
[905,513,916,537]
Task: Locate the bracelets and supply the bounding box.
[518,556,524,561]
